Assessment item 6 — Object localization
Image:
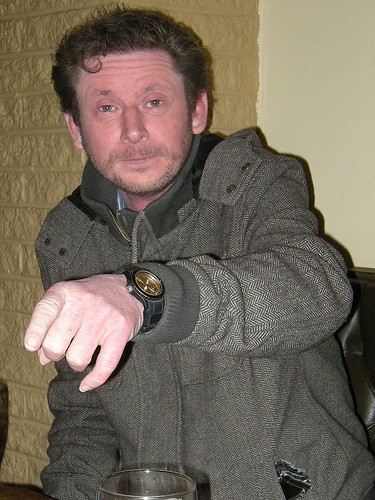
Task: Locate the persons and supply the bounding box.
[24,8,375,500]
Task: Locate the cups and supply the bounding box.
[97,469,197,500]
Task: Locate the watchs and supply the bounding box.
[119,266,165,335]
